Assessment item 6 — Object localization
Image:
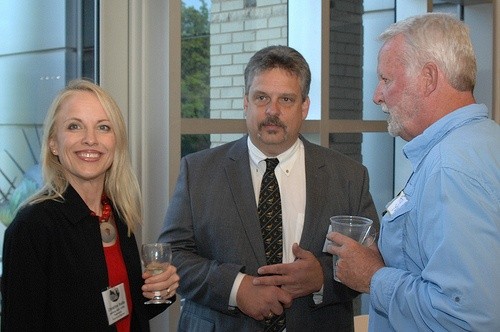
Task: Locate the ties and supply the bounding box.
[257,157,287,332]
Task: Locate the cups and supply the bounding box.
[330,215,373,282]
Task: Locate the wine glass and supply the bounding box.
[141,243,173,305]
